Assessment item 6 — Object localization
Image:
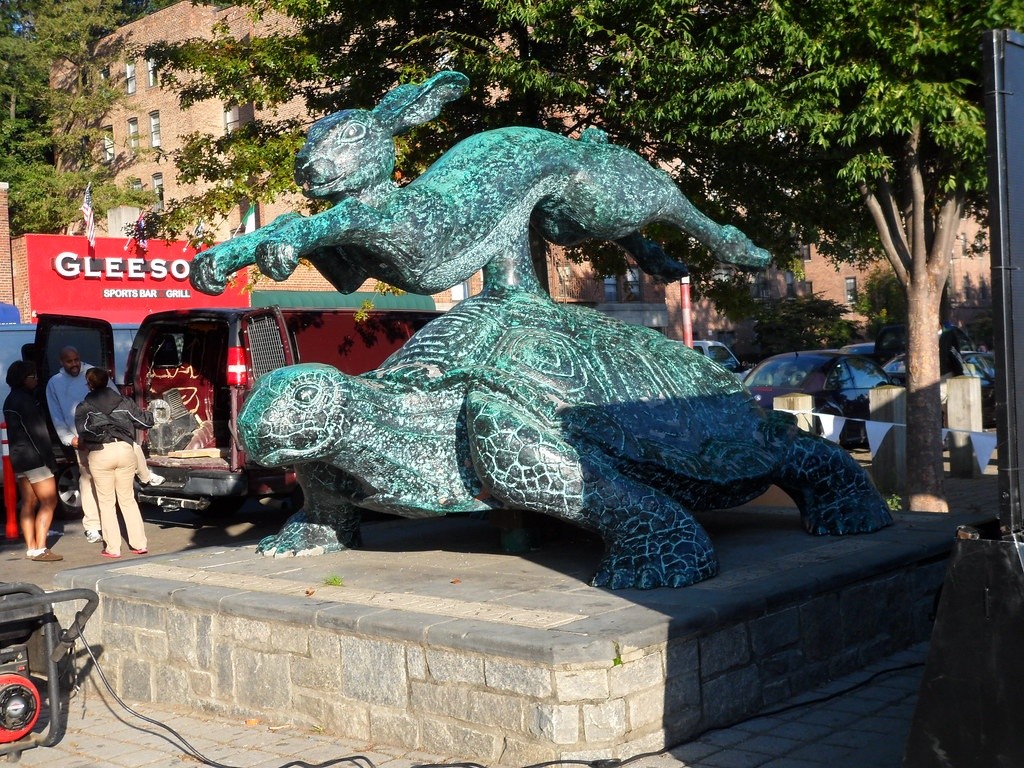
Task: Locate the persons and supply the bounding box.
[978,341,989,352]
[3,360,63,561]
[75,368,148,557]
[46,346,166,543]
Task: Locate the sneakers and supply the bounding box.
[138,472,166,486]
[86,529,103,544]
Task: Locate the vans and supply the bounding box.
[874,324,976,358]
[0,324,140,413]
[677,340,751,386]
[30,304,444,530]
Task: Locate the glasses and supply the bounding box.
[26,374,37,380]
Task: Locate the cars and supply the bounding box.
[838,342,998,428]
[742,351,901,446]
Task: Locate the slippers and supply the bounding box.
[28,547,64,561]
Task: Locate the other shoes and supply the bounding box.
[130,547,148,554]
[101,549,121,558]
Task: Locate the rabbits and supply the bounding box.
[190,71,772,294]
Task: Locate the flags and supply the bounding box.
[134,216,147,252]
[243,206,255,233]
[196,222,203,250]
[81,190,95,248]
